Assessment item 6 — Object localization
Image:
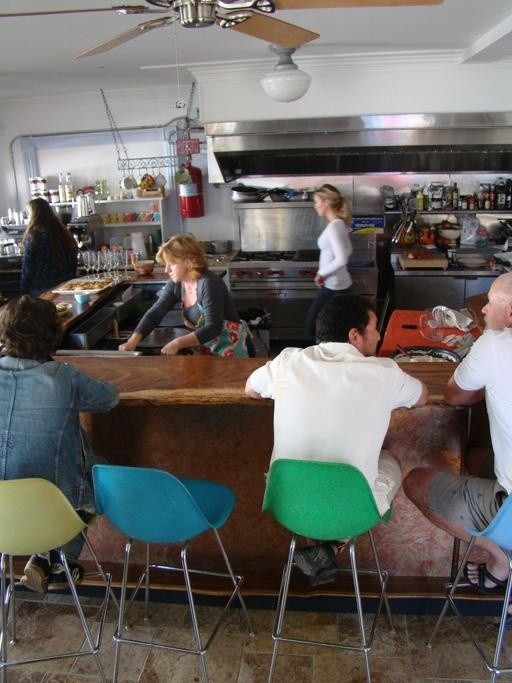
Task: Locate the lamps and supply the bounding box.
[260,38,313,104]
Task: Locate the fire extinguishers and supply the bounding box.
[176,145,204,218]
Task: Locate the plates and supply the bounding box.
[54,302,74,317]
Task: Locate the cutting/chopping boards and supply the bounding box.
[399,254,449,271]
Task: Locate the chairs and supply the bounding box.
[0,475,132,682]
[425,493,511,682]
[261,456,399,683]
[91,461,257,682]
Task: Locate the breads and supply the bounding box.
[61,278,113,291]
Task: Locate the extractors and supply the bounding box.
[203,111,511,184]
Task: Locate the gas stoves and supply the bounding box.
[227,248,321,280]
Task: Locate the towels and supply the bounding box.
[427,301,479,333]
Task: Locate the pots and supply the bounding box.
[135,322,194,349]
[228,181,322,202]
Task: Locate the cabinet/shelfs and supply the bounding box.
[39,193,175,248]
[388,250,507,310]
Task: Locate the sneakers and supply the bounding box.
[20,549,84,594]
[292,540,338,586]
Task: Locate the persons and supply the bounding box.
[304,184,353,338]
[118,234,256,358]
[245,293,429,588]
[18,199,79,299]
[1,294,120,594]
[402,271,511,632]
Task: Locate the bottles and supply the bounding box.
[66,172,74,202]
[76,187,96,218]
[447,243,457,259]
[106,191,127,200]
[58,172,67,203]
[100,228,161,258]
[95,179,110,200]
[29,177,60,204]
[382,175,512,210]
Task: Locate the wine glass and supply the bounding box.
[83,249,139,280]
[437,223,461,246]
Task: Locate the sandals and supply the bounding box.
[462,558,511,637]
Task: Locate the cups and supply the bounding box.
[76,304,89,313]
[101,202,160,224]
[119,171,168,191]
[1,238,25,255]
[0,208,30,226]
[75,292,91,304]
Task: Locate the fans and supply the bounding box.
[0,0,444,61]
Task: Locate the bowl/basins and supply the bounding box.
[450,245,503,269]
[389,344,462,363]
[133,260,156,276]
[211,240,232,253]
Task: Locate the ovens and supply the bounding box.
[229,283,323,341]
